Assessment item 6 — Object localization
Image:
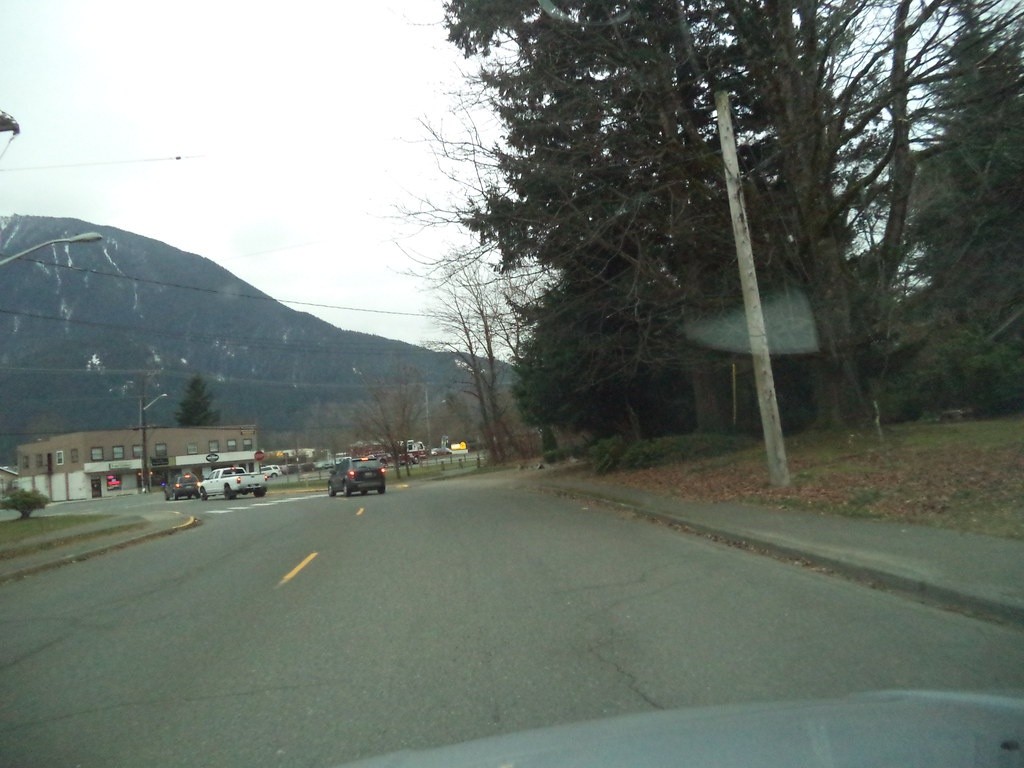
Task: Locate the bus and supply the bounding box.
[415,441,427,459]
[351,439,421,466]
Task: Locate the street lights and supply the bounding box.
[425,399,447,449]
[137,393,168,492]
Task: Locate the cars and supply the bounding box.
[430,448,451,456]
[279,456,353,475]
[261,464,282,478]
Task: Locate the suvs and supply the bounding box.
[327,454,385,497]
[163,471,201,501]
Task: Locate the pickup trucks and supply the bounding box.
[197,466,267,501]
